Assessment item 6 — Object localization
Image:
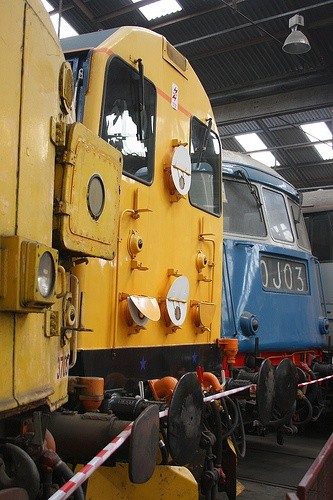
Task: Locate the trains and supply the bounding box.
[1,0,333,500]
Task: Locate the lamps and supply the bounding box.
[281,12,312,55]
